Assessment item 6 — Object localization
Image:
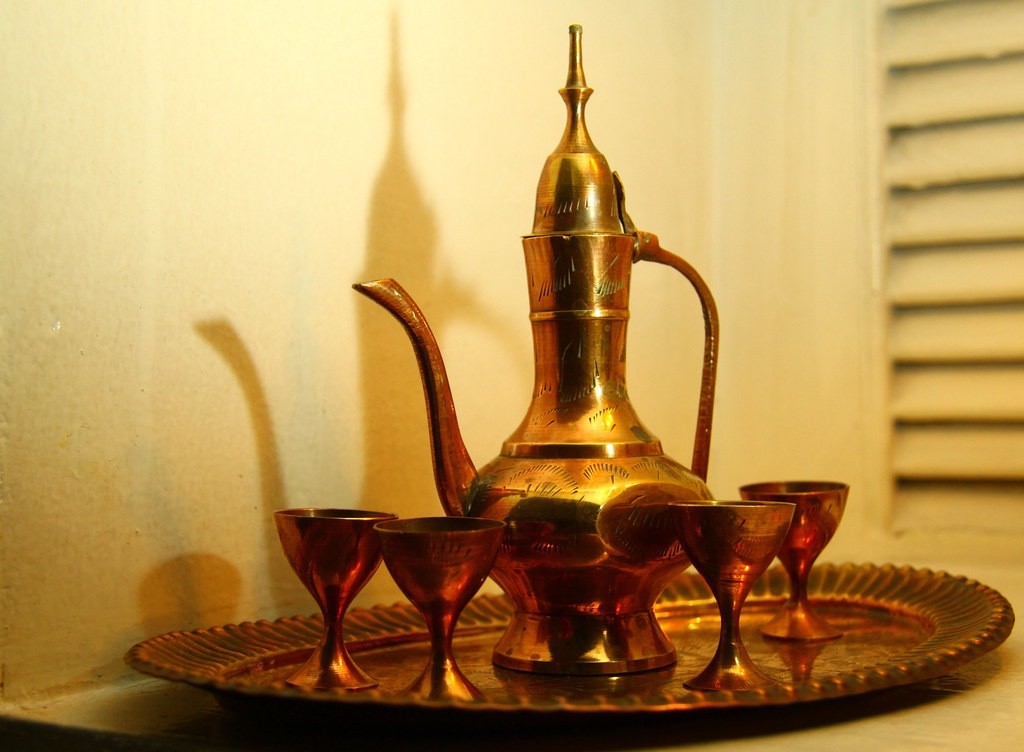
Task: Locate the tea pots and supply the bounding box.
[356,24,720,677]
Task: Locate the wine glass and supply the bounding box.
[374,518,506,705]
[739,481,850,642]
[669,501,796,693]
[275,507,397,695]
